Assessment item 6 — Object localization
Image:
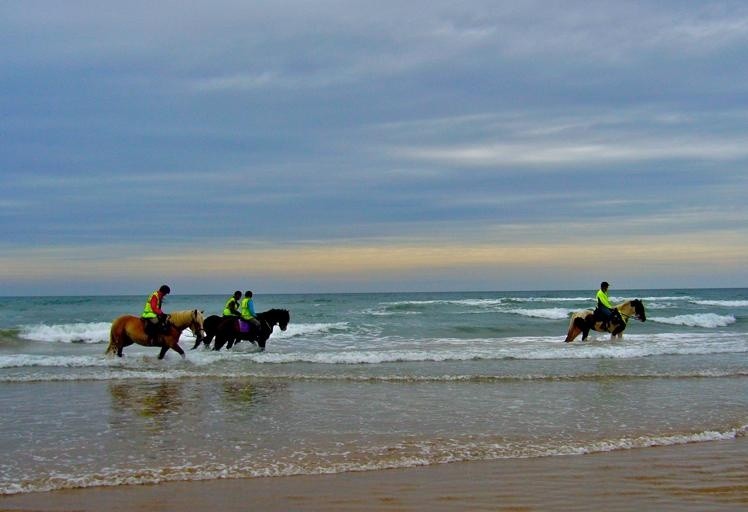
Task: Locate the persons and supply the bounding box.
[595,282,614,331]
[140,285,171,346]
[222,290,241,318]
[239,291,262,343]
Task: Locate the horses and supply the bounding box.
[105,308,205,361]
[564,298,647,343]
[191,315,257,351]
[212,308,290,352]
